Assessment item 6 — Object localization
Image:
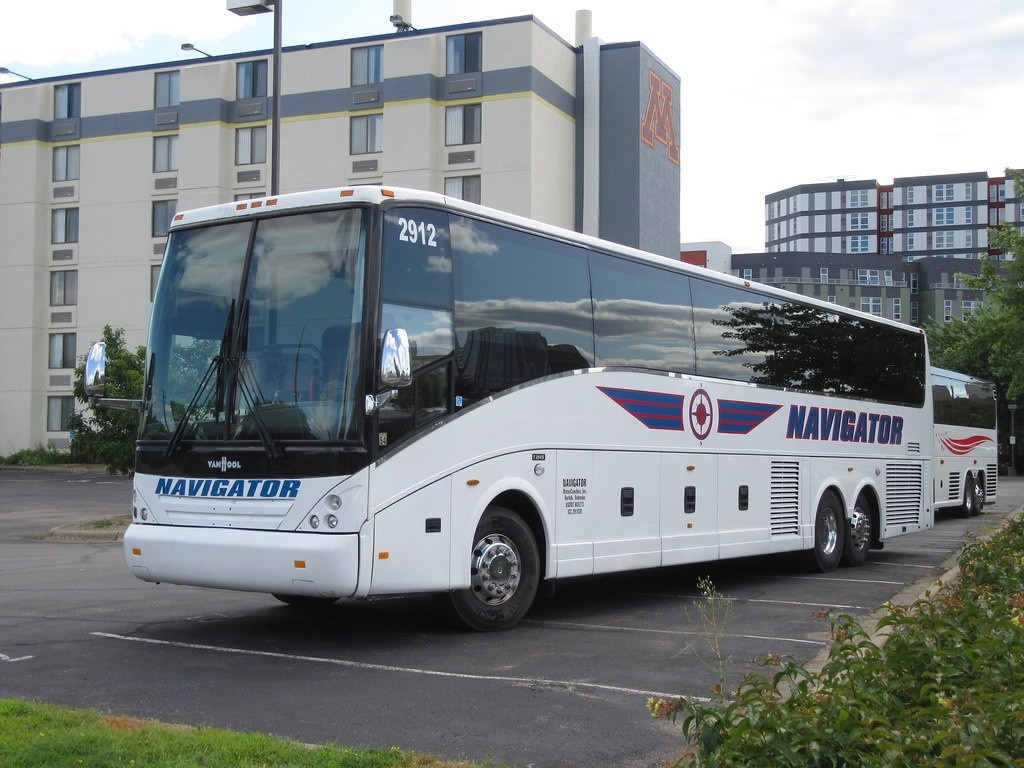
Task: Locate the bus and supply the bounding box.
[930,366,999,520]
[120,186,935,632]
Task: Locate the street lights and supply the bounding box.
[225,0,285,195]
